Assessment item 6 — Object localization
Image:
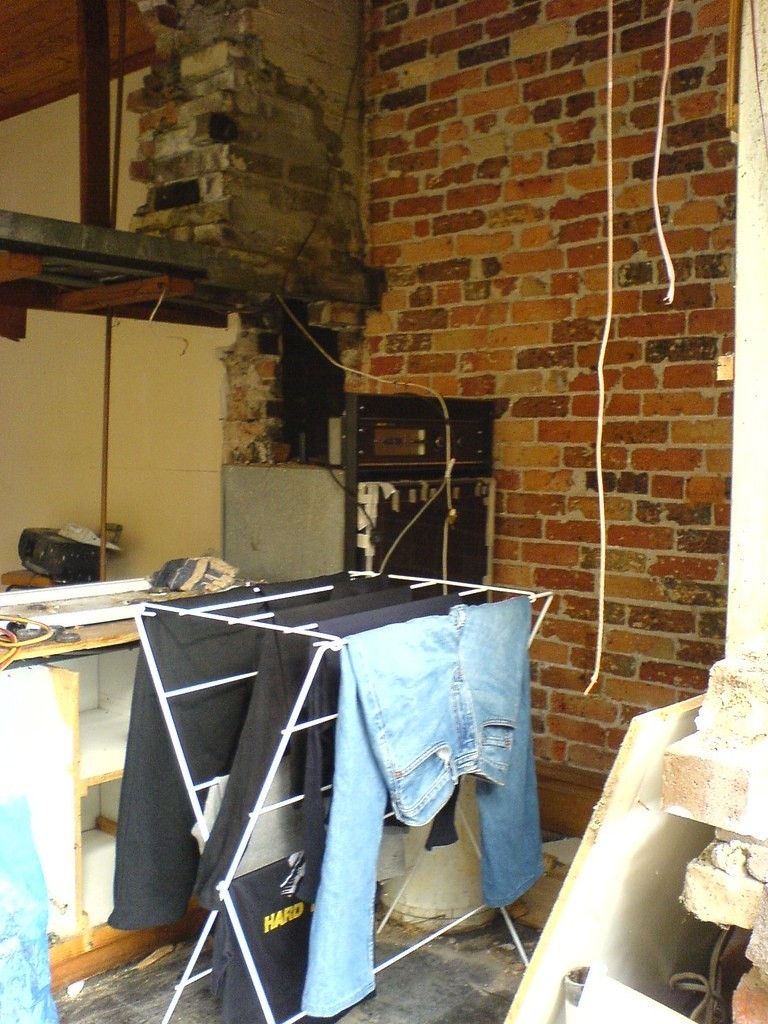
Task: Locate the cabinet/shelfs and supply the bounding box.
[0,619,215,991]
